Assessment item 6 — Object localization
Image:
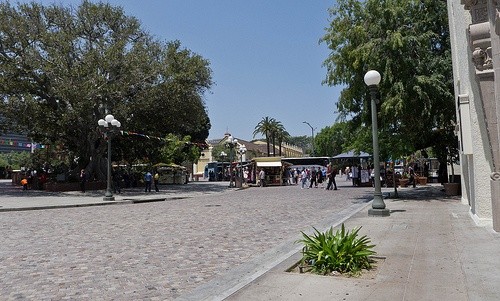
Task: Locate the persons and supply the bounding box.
[208,161,417,191]
[153,171,160,193]
[144,170,152,192]
[21,178,28,191]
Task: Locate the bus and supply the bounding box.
[204,162,250,181]
[247,157,284,187]
[279,156,332,179]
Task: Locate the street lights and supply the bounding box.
[97,114,121,200]
[364,70,390,217]
[302,121,314,141]
[226,134,238,188]
[236,144,247,190]
[219,150,227,182]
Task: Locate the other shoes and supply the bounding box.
[301,186,338,190]
